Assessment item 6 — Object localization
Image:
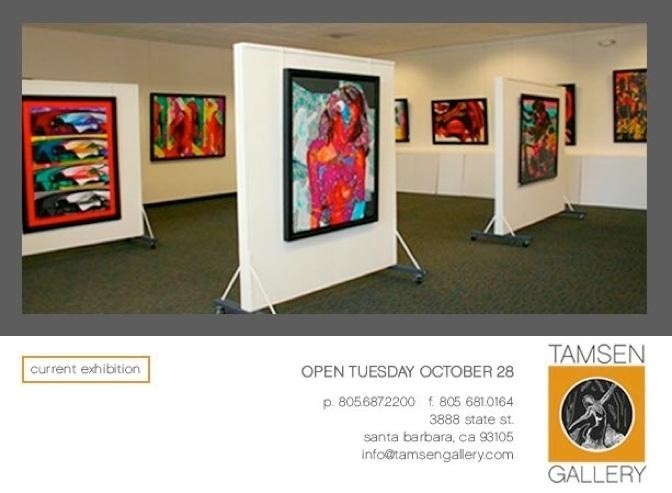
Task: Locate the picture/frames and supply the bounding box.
[431,96,488,146]
[558,85,576,147]
[519,93,560,186]
[612,68,646,143]
[283,68,378,242]
[395,99,409,143]
[21,95,122,235]
[149,92,226,162]
